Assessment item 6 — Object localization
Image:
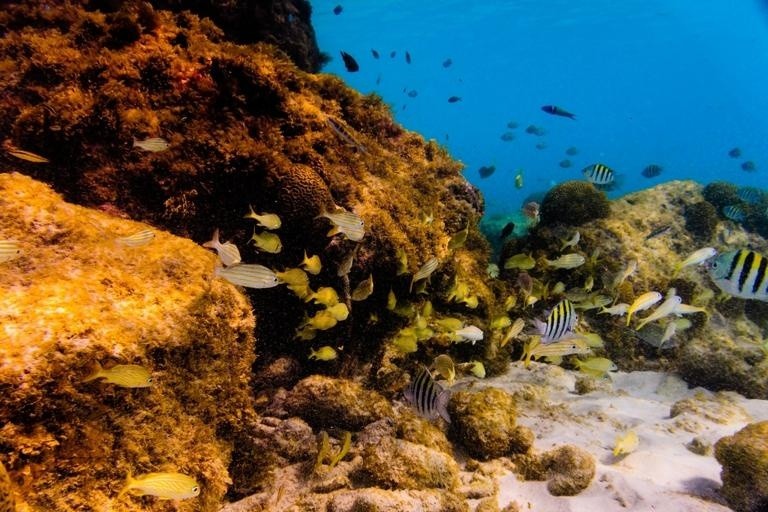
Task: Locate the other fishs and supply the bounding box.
[200,188,767,424]
